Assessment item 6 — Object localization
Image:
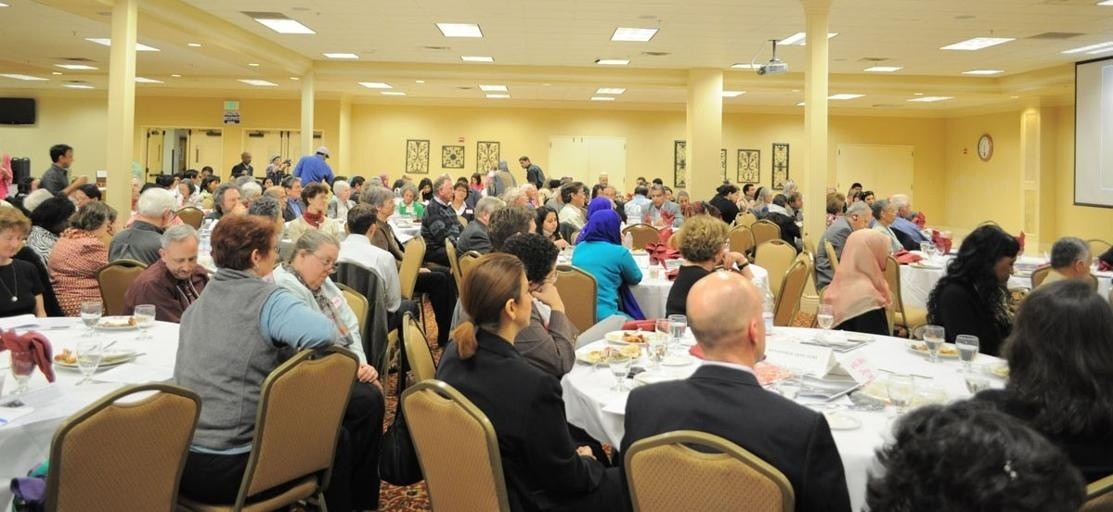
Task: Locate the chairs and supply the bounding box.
[624,429,794,511]
[0,181,1112,420]
[44,382,202,512]
[178,345,360,511]
[1081,474,1111,511]
[401,379,509,512]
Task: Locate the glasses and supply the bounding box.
[306,248,339,275]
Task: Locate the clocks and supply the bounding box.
[976,133,993,162]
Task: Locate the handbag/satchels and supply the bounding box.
[380,411,424,487]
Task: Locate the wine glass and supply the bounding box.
[885,376,913,423]
[76,300,158,393]
[955,336,980,378]
[665,314,690,353]
[609,356,633,399]
[924,327,944,367]
[814,307,833,338]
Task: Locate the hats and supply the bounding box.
[317,146,330,158]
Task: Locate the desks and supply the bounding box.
[1,315,180,511]
[560,324,1011,512]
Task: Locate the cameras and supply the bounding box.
[284,159,291,167]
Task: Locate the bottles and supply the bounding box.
[624,231,634,249]
[764,298,774,338]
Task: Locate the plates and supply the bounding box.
[906,261,942,270]
[575,343,640,369]
[604,329,659,346]
[45,355,132,375]
[984,361,1011,384]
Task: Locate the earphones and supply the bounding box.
[853,215,857,220]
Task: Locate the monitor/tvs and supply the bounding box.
[0,97,36,125]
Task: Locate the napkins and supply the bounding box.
[3,331,56,383]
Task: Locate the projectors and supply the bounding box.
[758,57,788,74]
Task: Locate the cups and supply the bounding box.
[920,241,947,261]
[1090,258,1101,273]
[649,261,662,280]
[410,211,417,220]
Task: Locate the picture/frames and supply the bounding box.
[673,140,686,188]
[441,144,465,169]
[404,139,430,174]
[476,141,500,177]
[771,143,789,191]
[720,149,727,183]
[736,149,761,184]
